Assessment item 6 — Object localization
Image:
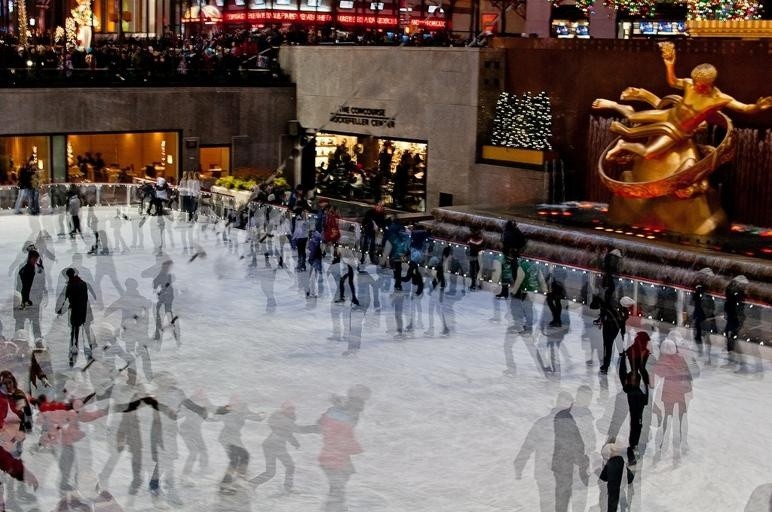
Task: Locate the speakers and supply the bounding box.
[287,120,302,136]
[439,192,452,207]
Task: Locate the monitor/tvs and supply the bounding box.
[387,32,393,39]
[37,159,43,170]
[197,0,444,14]
[402,35,408,42]
[676,22,684,31]
[575,25,589,35]
[639,21,653,32]
[554,26,568,34]
[658,23,671,32]
[423,33,432,39]
[166,154,173,165]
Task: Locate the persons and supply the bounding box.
[591,41,772,161]
